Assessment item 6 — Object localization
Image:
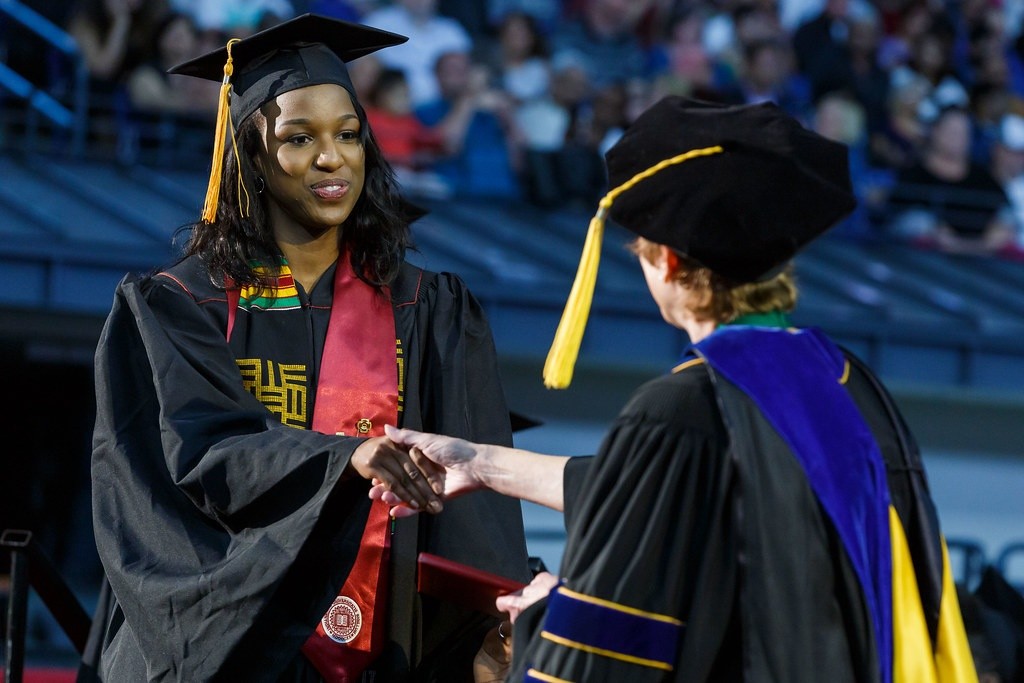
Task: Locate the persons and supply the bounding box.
[369,95,978,683]
[78,14,528,683]
[69,0,1024,256]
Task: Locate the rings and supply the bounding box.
[498,624,504,638]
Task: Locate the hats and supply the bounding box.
[542,95,858,391]
[165,14,410,224]
